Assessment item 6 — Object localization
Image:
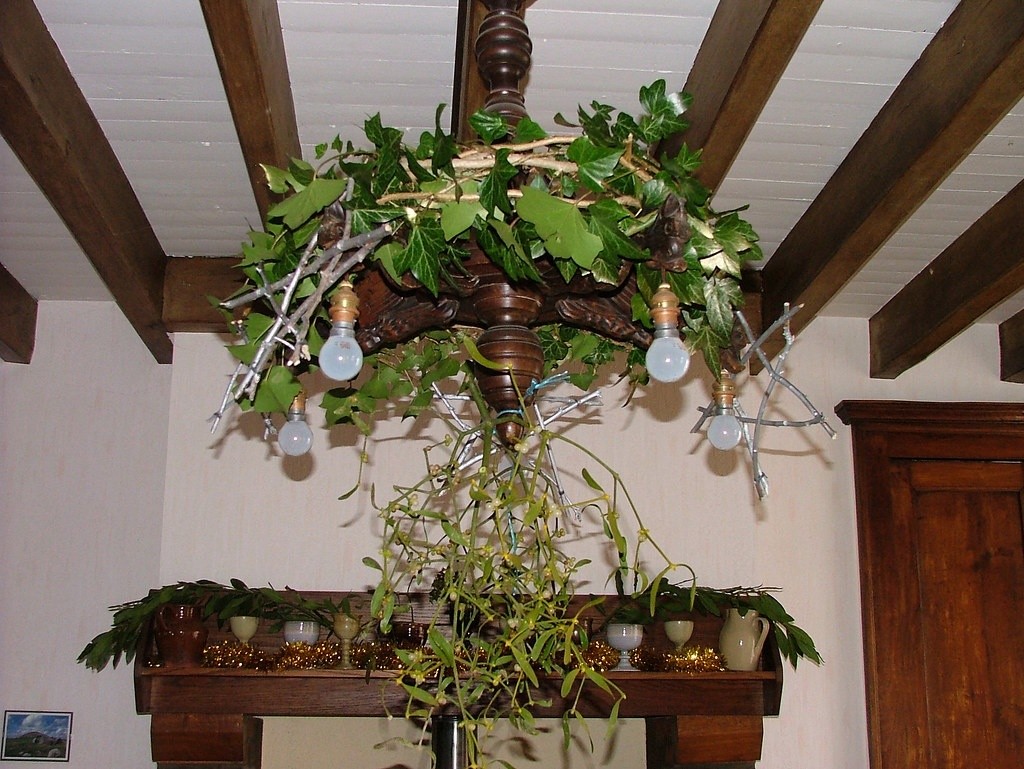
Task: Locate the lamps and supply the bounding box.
[212,11,837,502]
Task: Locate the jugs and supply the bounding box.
[159,603,208,668]
[718,607,769,671]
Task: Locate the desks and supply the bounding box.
[133,576,785,769]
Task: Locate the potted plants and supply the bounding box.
[75,578,365,674]
[590,578,826,671]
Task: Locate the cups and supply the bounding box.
[285,620,320,645]
[391,621,429,648]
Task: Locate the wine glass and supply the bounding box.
[665,621,694,652]
[606,624,644,672]
[230,617,259,645]
[332,614,361,670]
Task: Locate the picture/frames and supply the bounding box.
[1,710,74,763]
